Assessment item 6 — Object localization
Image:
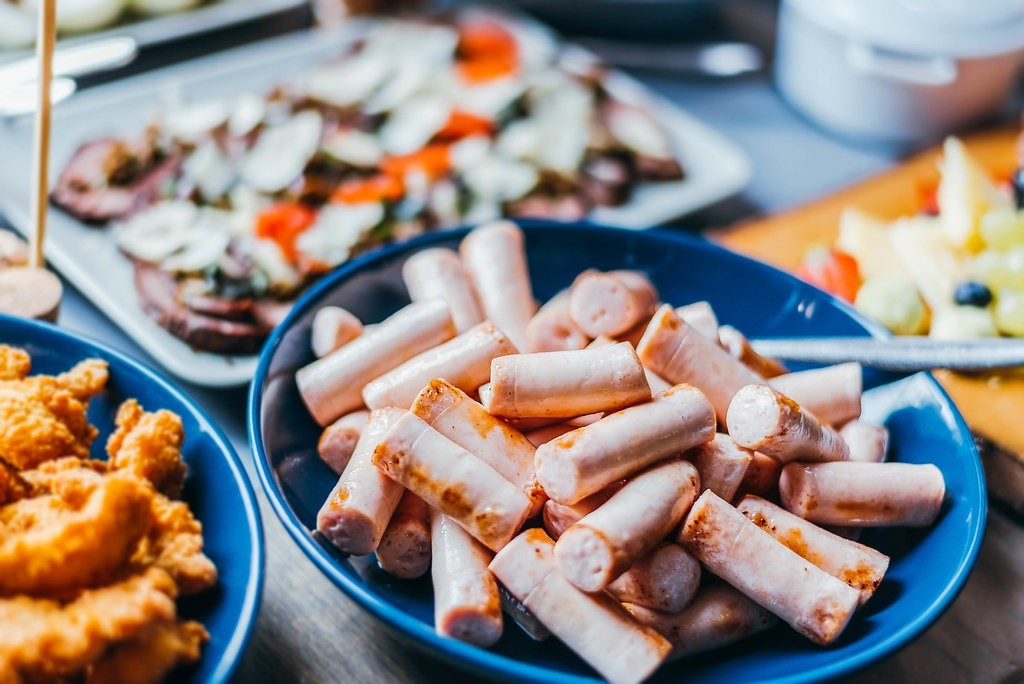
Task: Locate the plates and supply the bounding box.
[243,215,988,684]
[0,312,269,684]
[703,119,1023,462]
[0,0,752,388]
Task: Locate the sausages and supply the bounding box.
[300,219,945,684]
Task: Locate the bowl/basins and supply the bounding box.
[772,0,1024,147]
